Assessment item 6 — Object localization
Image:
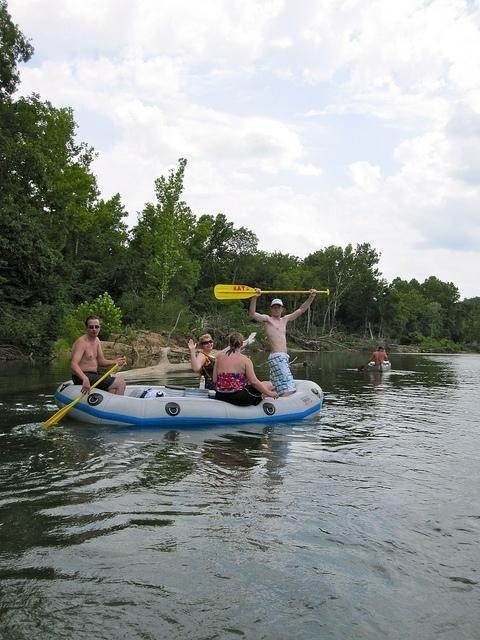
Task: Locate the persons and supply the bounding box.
[369,346,389,366]
[187,287,317,406]
[70,315,127,396]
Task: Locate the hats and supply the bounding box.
[270,298,284,307]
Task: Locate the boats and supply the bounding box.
[368,359,392,370]
[54,379,324,427]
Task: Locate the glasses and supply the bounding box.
[88,325,100,329]
[201,340,214,345]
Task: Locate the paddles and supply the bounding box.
[43,356,127,426]
[358,356,375,370]
[214,284,331,300]
